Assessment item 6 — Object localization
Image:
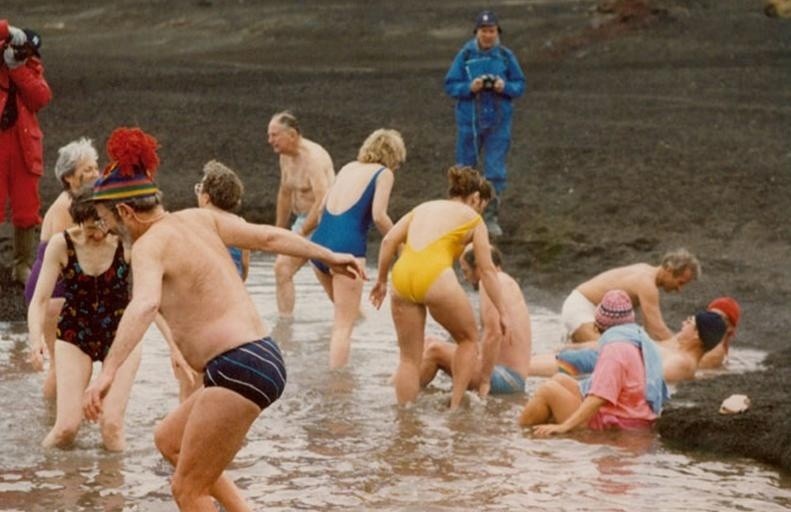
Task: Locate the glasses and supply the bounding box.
[193,183,209,203]
[93,215,110,230]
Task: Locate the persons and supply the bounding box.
[27,187,198,453]
[561,250,700,343]
[0,20,56,286]
[309,128,406,370]
[528,312,726,382]
[268,111,366,317]
[368,167,518,411]
[84,127,365,512]
[519,290,671,438]
[419,242,531,397]
[178,160,250,407]
[553,296,741,369]
[25,137,101,399]
[443,11,526,236]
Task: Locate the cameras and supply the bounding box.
[481,76,497,91]
[3,35,35,62]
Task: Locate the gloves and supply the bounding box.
[5,24,25,48]
[3,48,25,71]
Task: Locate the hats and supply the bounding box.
[594,291,634,327]
[473,11,500,34]
[15,29,44,56]
[694,311,726,356]
[92,160,158,198]
[708,297,741,327]
